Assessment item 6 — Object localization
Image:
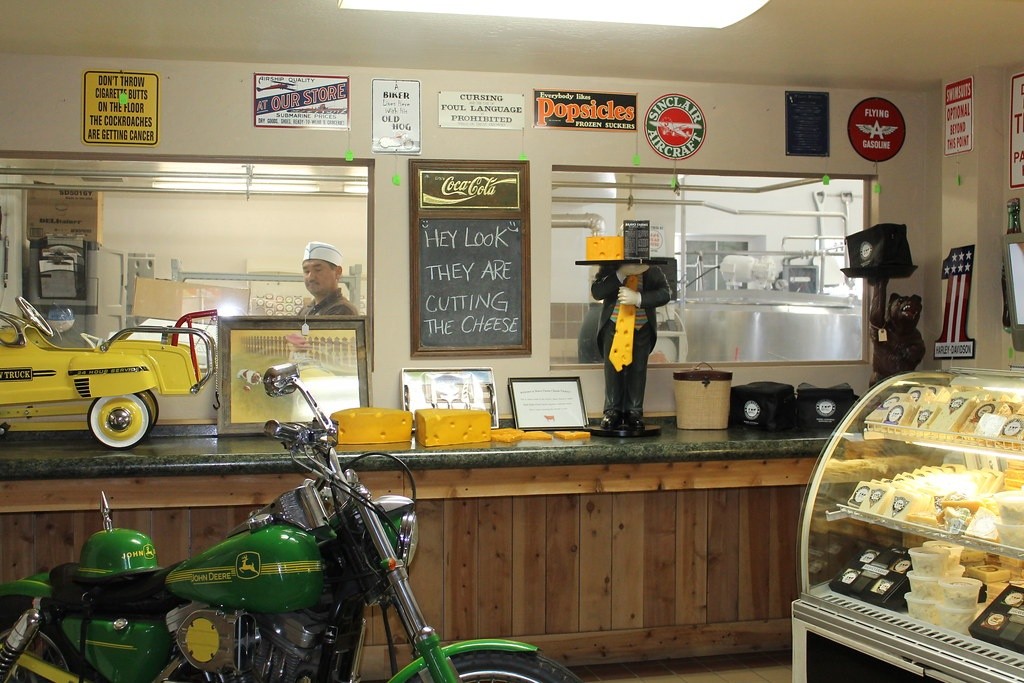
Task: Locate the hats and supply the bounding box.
[303,241,343,267]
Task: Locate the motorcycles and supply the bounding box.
[0,362,587,683]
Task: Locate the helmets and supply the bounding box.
[67,490,162,577]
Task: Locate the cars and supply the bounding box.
[0,294,219,454]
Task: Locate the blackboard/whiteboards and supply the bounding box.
[412,156,532,359]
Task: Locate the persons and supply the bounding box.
[592,259,671,431]
[295,242,357,315]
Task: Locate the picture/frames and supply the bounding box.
[399,367,500,430]
[217,315,373,436]
[508,377,590,431]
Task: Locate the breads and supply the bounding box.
[847,384,1024,605]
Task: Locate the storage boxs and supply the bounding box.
[26,181,103,244]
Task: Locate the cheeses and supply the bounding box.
[328,406,413,445]
[415,409,591,446]
